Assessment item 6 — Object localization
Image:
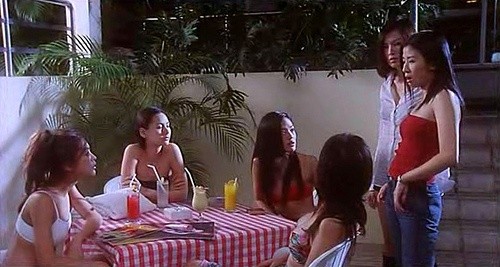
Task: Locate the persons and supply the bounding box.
[120,105,188,202]
[368,20,425,267]
[251,111,319,222]
[184,133,374,267]
[0,128,111,267]
[385,30,467,267]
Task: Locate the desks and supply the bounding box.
[65,199,299,267]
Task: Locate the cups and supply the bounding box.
[157,180,169,208]
[126,190,142,225]
[223,180,239,213]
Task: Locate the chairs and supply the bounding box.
[308,232,360,267]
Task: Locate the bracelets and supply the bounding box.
[397,175,409,185]
[388,180,394,189]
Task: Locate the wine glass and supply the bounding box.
[191,186,207,222]
